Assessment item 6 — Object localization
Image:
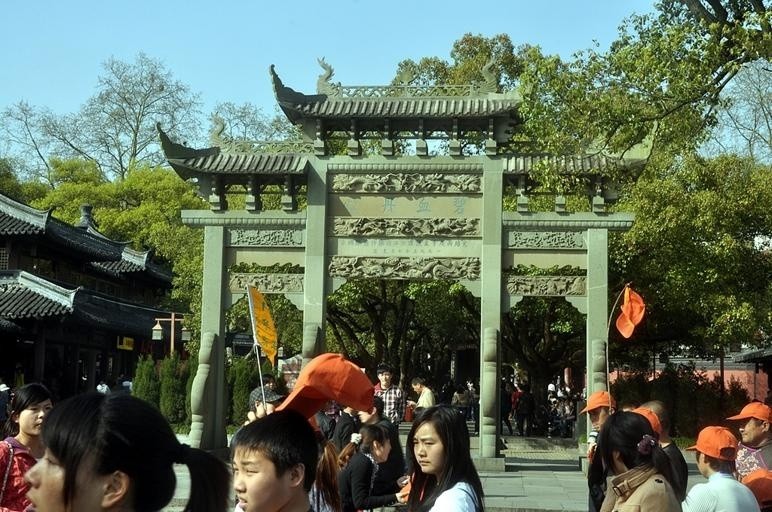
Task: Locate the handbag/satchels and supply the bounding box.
[403,405,414,423]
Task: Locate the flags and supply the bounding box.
[245,283,278,368]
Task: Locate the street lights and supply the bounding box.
[151,313,190,359]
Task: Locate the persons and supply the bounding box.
[0,361,485,512]
[724,400,772,512]
[680,426,760,511]
[500,379,576,441]
[579,389,689,511]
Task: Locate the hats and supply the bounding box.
[726,401,772,426]
[577,390,619,417]
[248,383,287,407]
[630,406,663,436]
[615,284,647,340]
[684,424,740,464]
[739,468,772,510]
[271,352,377,426]
